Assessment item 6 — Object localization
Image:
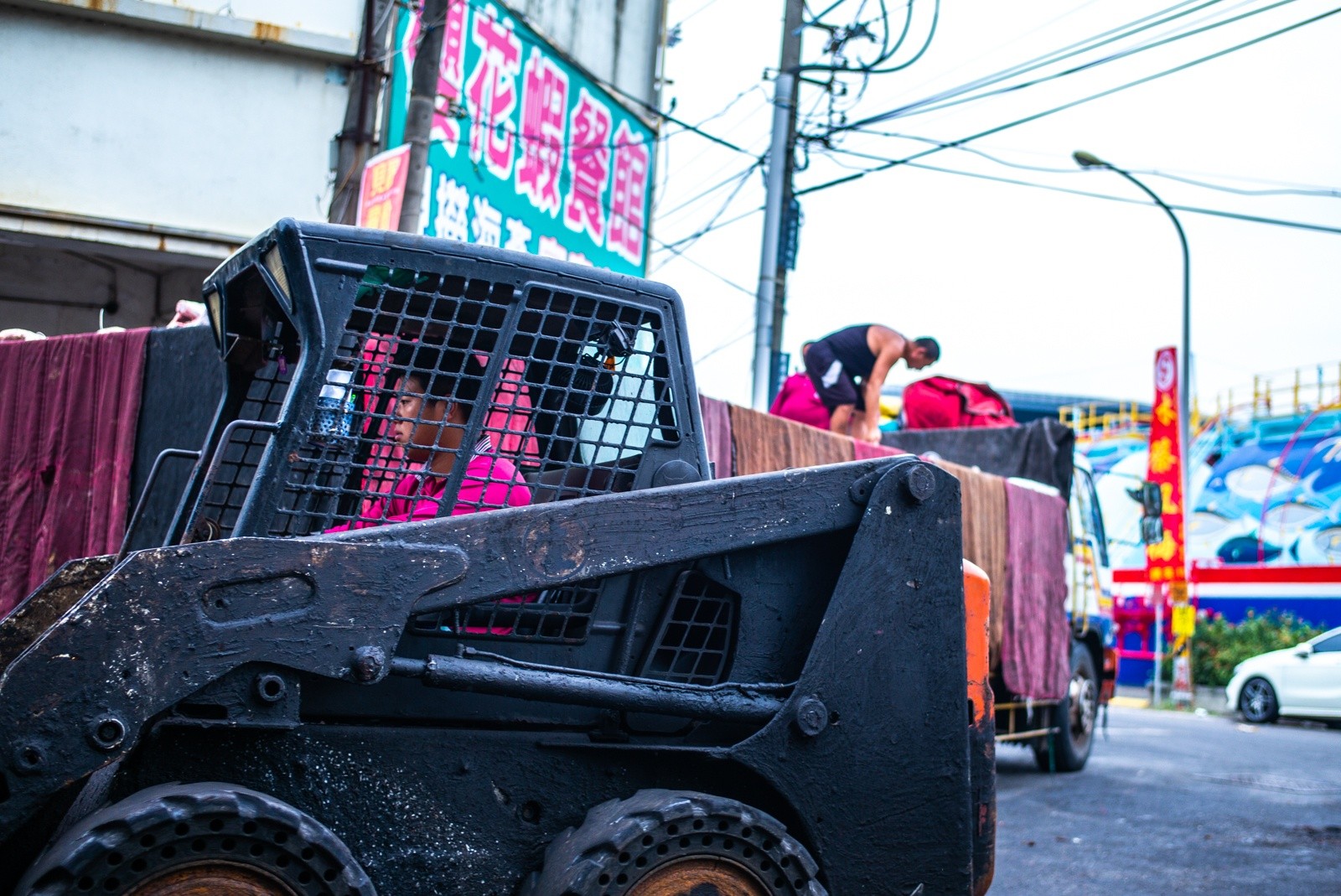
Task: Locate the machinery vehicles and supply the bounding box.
[0,217,997,896]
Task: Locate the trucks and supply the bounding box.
[696,395,1116,772]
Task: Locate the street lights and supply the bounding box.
[1073,150,1195,711]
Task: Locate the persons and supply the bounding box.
[323,338,541,637]
[805,324,939,444]
[767,342,855,430]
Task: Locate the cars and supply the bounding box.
[1224,626,1341,724]
